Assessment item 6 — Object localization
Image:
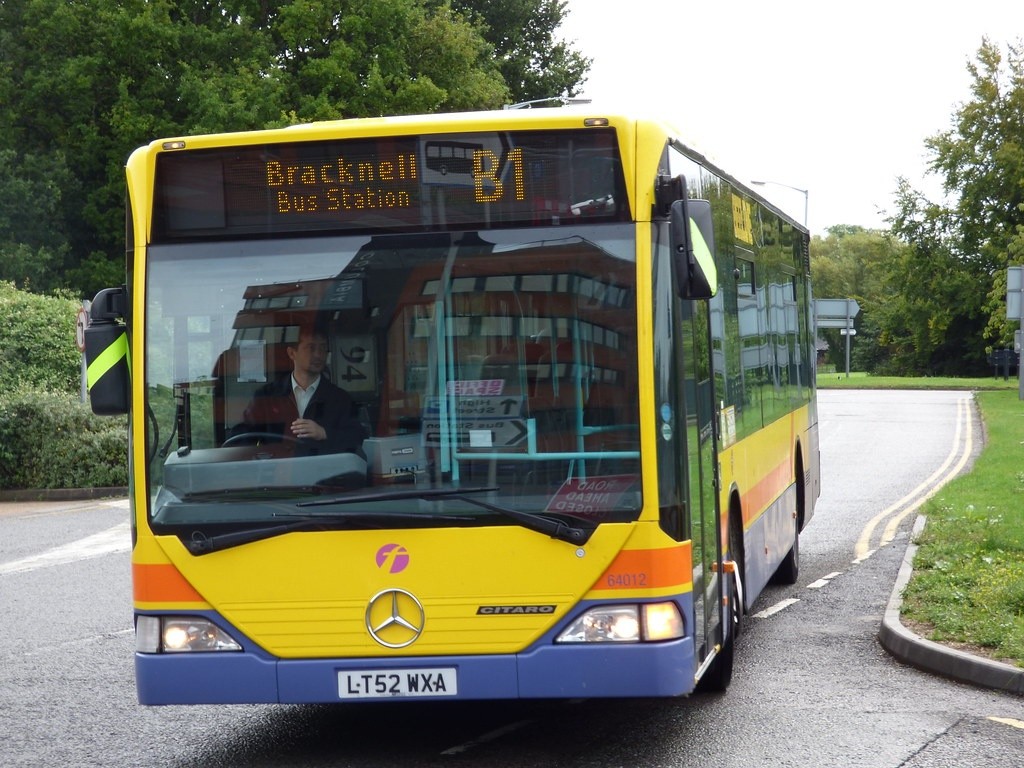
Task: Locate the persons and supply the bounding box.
[230,329,361,445]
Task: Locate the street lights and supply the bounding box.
[501,97,591,109]
[748,177,810,229]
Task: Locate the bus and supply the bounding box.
[82,109,825,707]
